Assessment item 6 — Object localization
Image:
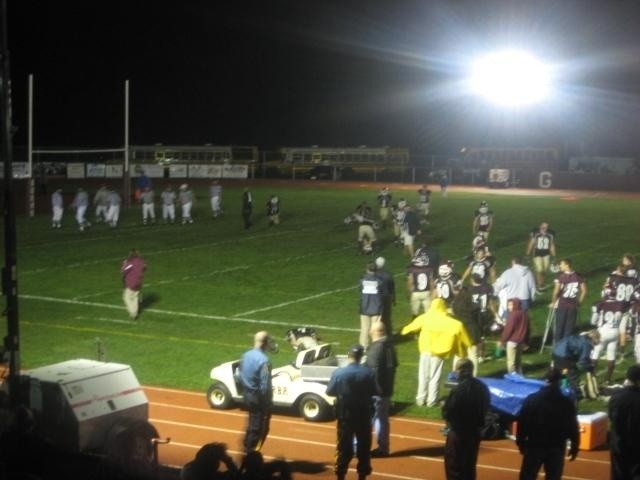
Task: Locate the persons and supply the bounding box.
[325,344,383,479]
[180,442,238,480]
[516,369,579,479]
[608,364,640,480]
[344,172,640,407]
[238,450,291,479]
[365,322,400,457]
[240,330,273,453]
[441,359,490,480]
[119,248,147,320]
[49,169,281,233]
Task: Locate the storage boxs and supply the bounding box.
[571,410,610,452]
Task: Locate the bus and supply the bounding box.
[263,145,410,178]
[563,143,640,173]
[454,145,559,173]
[129,142,259,175]
